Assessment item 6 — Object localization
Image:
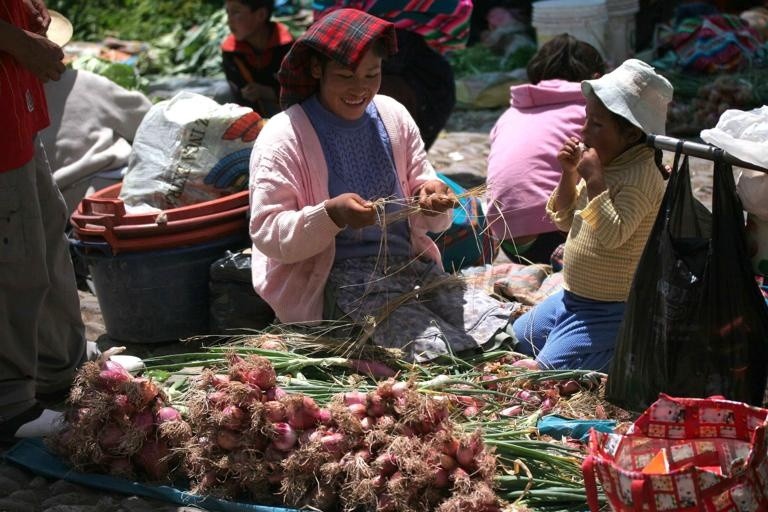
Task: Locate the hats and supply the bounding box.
[581,59,673,139]
[45,9,73,48]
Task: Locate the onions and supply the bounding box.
[51,358,580,512]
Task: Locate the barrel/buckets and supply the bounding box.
[68,182,250,348]
[530,0,640,69]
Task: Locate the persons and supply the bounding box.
[43,8,155,290]
[513,58,672,373]
[250,6,518,362]
[0,1,145,441]
[222,0,295,120]
[486,34,605,265]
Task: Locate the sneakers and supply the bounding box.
[108,355,147,377]
[0,403,68,438]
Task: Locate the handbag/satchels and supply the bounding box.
[580,393,766,512]
